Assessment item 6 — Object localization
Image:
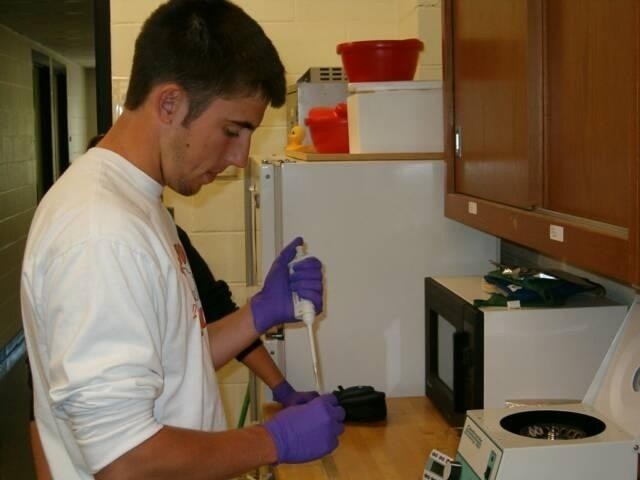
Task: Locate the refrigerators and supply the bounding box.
[248,158,499,404]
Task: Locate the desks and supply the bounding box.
[260,394,463,480]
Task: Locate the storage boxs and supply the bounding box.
[345,81,445,155]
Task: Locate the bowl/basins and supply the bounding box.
[333,38,425,86]
[304,106,353,157]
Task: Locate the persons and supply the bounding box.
[19,0,347,480]
[25,134,321,480]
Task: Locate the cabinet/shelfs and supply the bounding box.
[437,1,639,289]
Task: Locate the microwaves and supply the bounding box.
[424,269,629,440]
